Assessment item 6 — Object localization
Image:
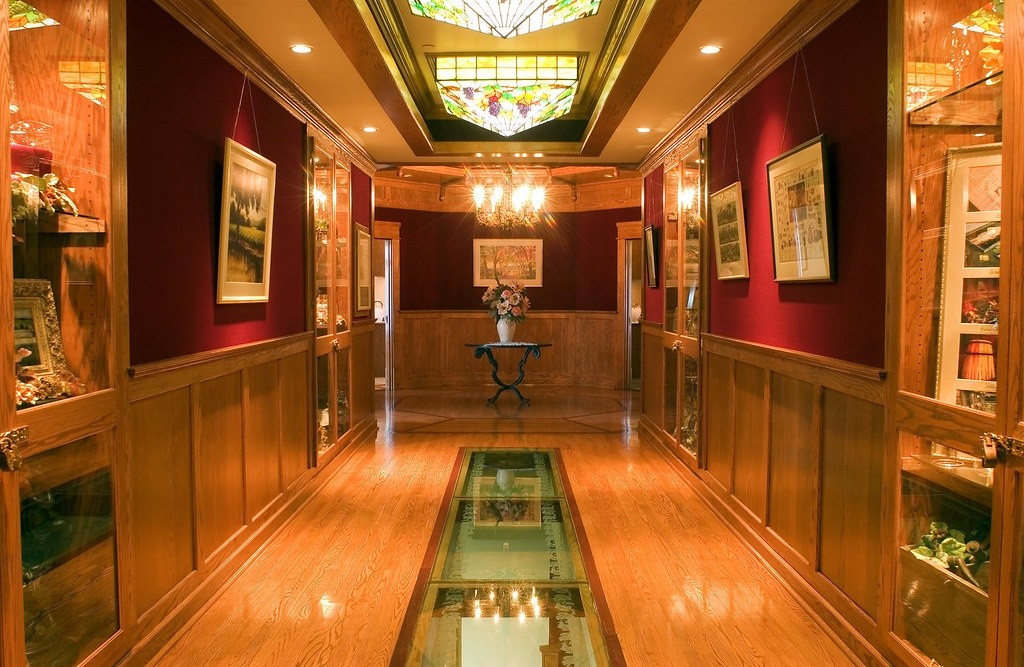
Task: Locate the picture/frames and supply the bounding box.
[214,137,276,305]
[929,142,1003,460]
[766,133,837,284]
[644,224,659,287]
[13,278,67,404]
[710,180,750,280]
[353,222,372,318]
[472,237,545,289]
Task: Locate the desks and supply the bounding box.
[464,341,553,407]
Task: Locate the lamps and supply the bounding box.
[473,560,540,628]
[472,163,545,232]
[407,0,602,39]
[434,56,579,138]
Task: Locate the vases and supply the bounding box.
[496,315,517,343]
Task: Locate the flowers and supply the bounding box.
[482,280,532,322]
